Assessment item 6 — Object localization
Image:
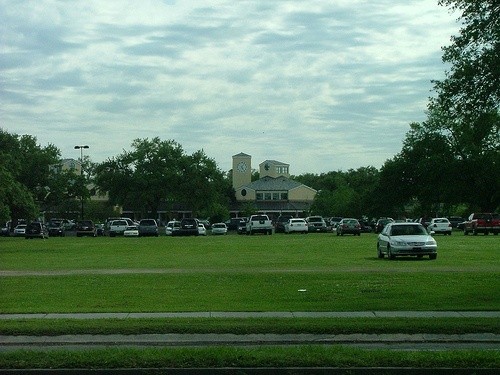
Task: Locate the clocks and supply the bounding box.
[236,161,247,173]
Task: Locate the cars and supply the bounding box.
[1,212,500,239]
[376,221,438,259]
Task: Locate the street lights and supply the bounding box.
[73,144,90,221]
